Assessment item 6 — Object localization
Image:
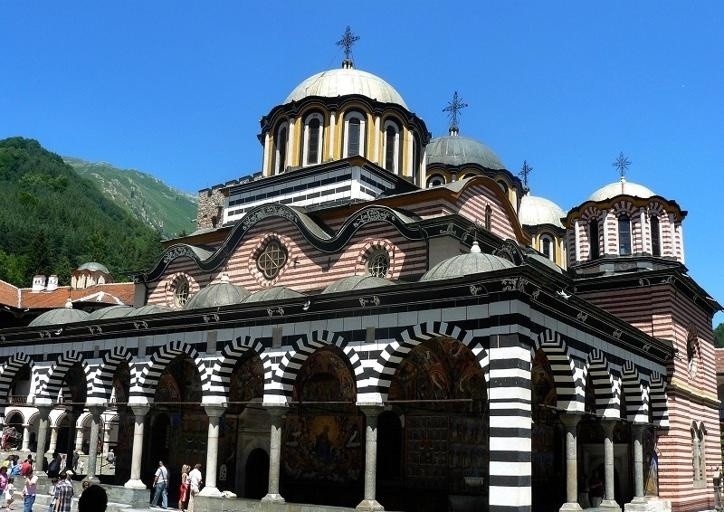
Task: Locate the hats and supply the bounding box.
[65,470,74,475]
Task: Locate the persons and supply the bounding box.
[317,423,339,463]
[0,425,107,512]
[177,463,190,512]
[587,468,604,506]
[186,462,203,512]
[107,448,117,465]
[149,459,168,509]
[577,472,589,508]
